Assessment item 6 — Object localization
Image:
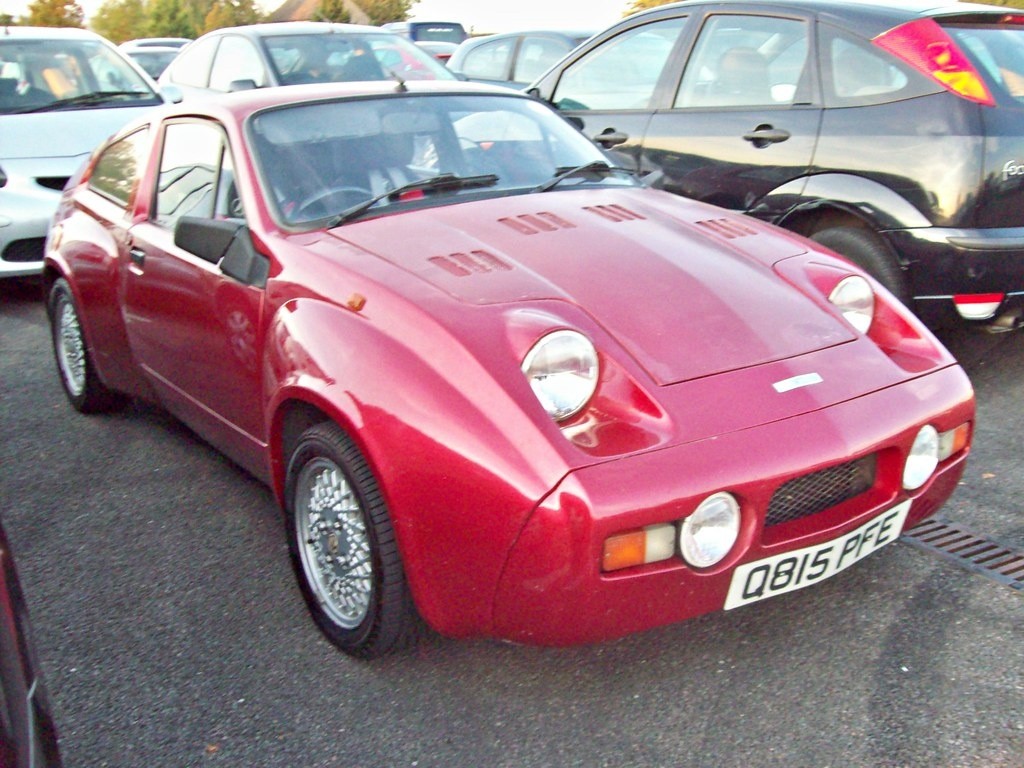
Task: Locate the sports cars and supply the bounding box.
[44,95,980,663]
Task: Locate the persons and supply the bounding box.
[712,46,778,105]
[306,44,332,77]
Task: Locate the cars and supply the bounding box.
[115,35,193,78]
[154,22,460,102]
[0,513,56,768]
[514,0,1024,353]
[442,28,600,90]
[377,21,467,61]
[0,23,183,284]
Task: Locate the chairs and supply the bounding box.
[834,45,893,92]
[717,47,770,104]
